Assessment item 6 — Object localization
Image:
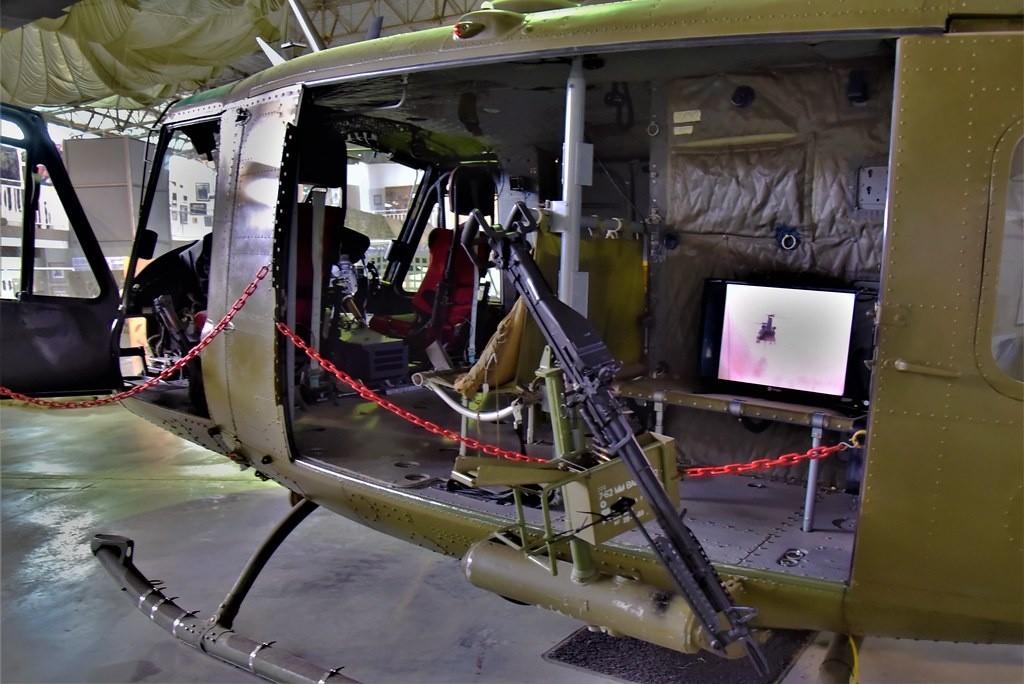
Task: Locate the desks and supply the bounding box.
[608,367,856,532]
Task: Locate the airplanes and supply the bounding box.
[0,0,1024,684]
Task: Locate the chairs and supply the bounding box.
[272,202,336,370]
[367,228,495,368]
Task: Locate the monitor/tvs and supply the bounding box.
[694,278,879,413]
